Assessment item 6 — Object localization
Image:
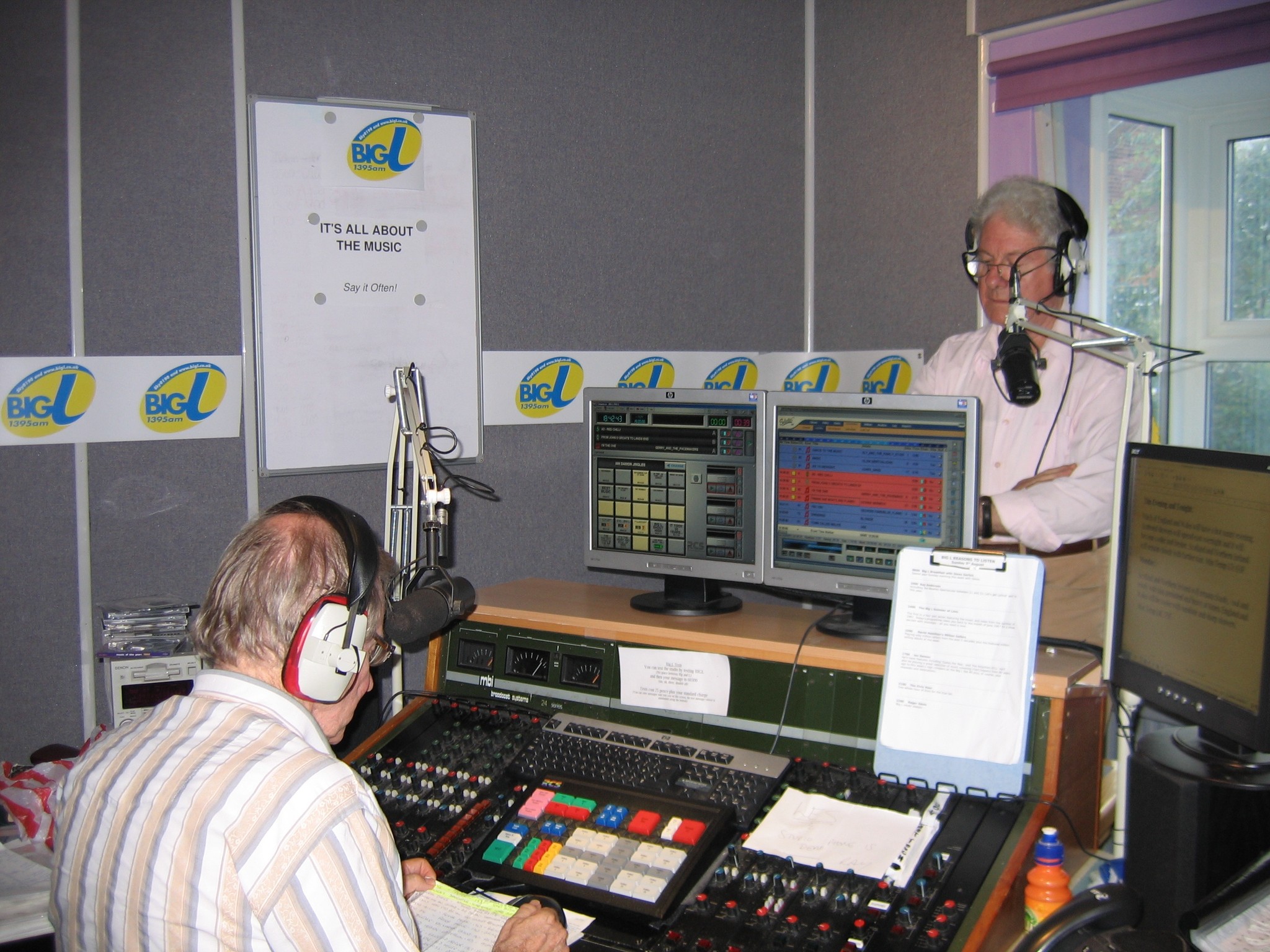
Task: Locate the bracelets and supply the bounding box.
[980,495,993,539]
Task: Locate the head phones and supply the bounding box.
[282,497,379,702]
[961,187,1088,298]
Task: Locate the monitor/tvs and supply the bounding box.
[1108,438,1269,771]
[582,386,762,619]
[766,396,981,639]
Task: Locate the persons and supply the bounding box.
[48,497,570,952]
[910,175,1144,760]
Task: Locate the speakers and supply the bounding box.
[1123,748,1269,935]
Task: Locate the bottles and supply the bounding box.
[1024,827,1076,952]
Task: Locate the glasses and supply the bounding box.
[365,630,397,668]
[967,252,1052,284]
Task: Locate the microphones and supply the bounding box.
[385,577,477,644]
[995,324,1042,405]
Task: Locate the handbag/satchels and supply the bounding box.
[1,724,108,853]
